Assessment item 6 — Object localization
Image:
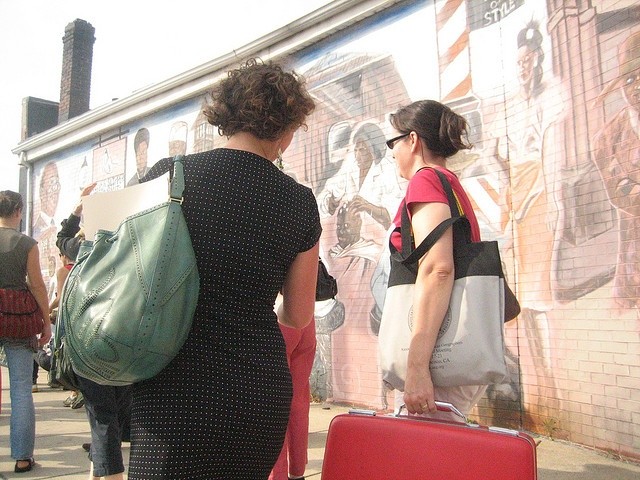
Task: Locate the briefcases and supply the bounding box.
[321,401,537,480]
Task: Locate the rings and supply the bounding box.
[422,404,428,409]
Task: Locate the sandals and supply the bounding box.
[16,458,34,472]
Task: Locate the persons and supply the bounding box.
[31,298,58,392]
[49,253,85,409]
[57,183,130,480]
[0,190,52,473]
[318,123,395,410]
[335,201,362,249]
[376,102,506,423]
[129,65,322,480]
[33,163,61,255]
[590,32,640,310]
[127,128,154,187]
[269,262,337,480]
[495,27,566,420]
[45,256,57,306]
[168,121,187,157]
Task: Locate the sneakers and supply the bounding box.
[63,396,75,406]
[71,393,85,409]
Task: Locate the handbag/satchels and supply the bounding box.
[59,157,198,387]
[504,279,521,322]
[0,288,42,339]
[316,257,337,300]
[379,240,510,391]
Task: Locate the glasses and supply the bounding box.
[389,132,411,150]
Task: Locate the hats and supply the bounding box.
[515,17,546,48]
[589,30,640,110]
